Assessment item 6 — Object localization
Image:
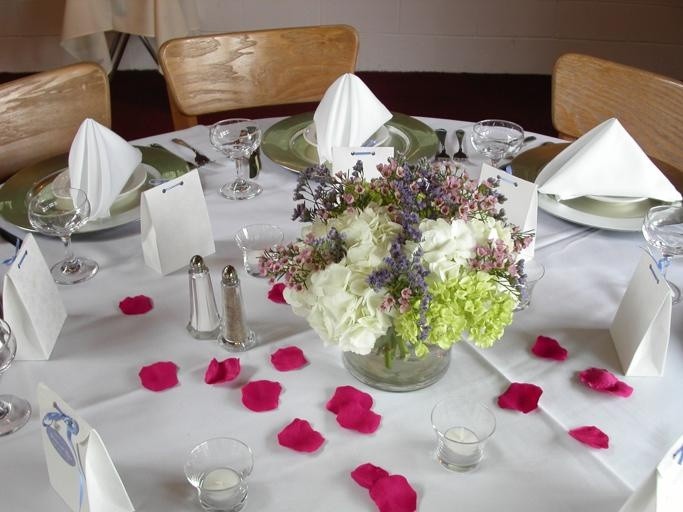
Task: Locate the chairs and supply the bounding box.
[158,23,360,127]
[1,55,113,186]
[548,51,683,174]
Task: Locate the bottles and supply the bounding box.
[185,255,224,342]
[217,264,256,354]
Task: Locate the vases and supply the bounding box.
[335,324,451,393]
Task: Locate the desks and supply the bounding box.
[58,2,188,80]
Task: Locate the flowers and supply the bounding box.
[256,151,536,358]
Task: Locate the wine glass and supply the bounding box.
[0,318,33,440]
[471,117,525,172]
[208,116,265,202]
[640,204,682,306]
[26,186,100,286]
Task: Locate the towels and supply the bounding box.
[60,115,143,225]
[532,115,680,205]
[301,70,395,178]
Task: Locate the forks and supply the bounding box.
[453,128,470,163]
[171,136,217,169]
[435,125,453,166]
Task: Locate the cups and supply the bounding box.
[428,393,497,474]
[232,222,285,279]
[501,256,545,311]
[180,435,258,512]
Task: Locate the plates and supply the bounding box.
[514,143,682,234]
[259,112,439,177]
[1,146,189,235]
[582,190,648,207]
[53,166,147,201]
[303,120,390,149]
